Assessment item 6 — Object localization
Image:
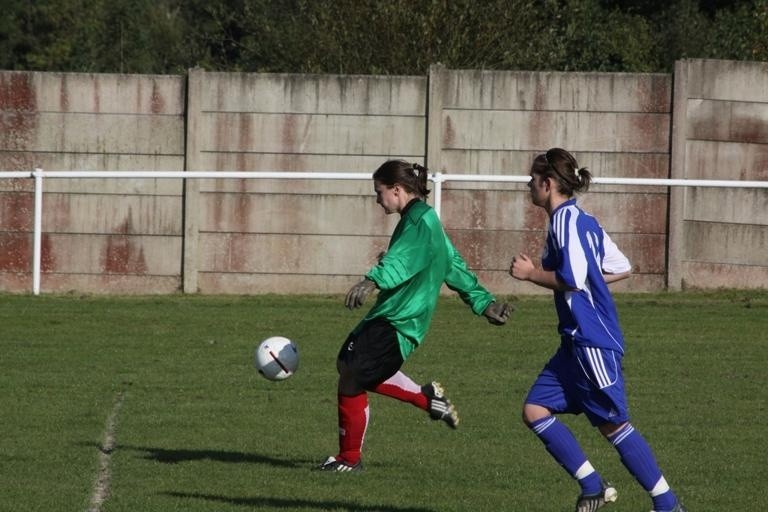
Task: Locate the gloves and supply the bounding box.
[484,301,515,326]
[343,278,377,311]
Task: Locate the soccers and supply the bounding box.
[254,336,300,382]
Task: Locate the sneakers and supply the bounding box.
[420,380,460,431]
[575,479,618,512]
[310,456,364,473]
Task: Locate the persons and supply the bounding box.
[313,158,516,475]
[506,145,689,512]
